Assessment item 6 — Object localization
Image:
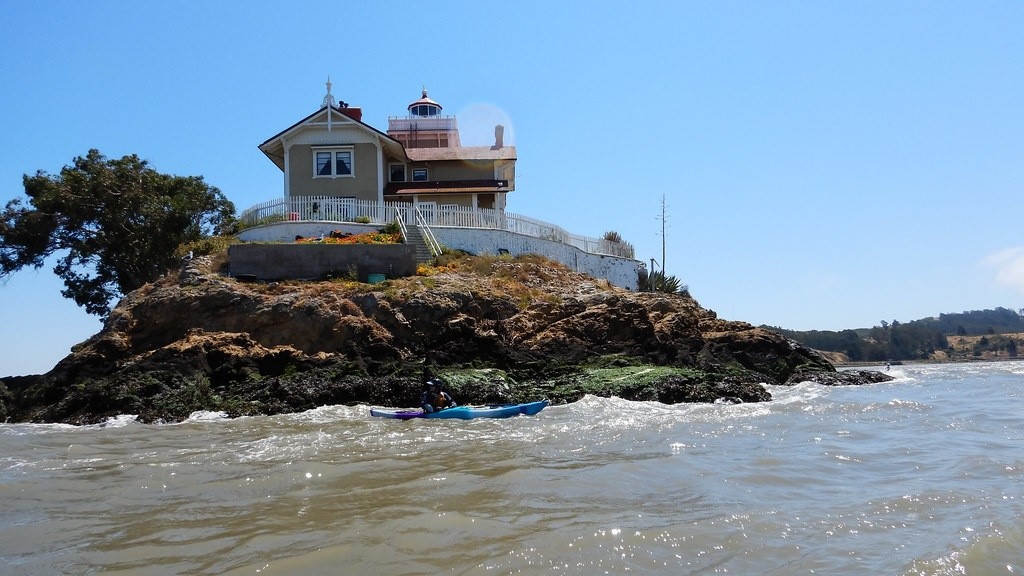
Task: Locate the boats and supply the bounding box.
[370,399,548,420]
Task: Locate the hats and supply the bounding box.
[426,378,441,387]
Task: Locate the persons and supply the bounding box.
[421,379,457,412]
